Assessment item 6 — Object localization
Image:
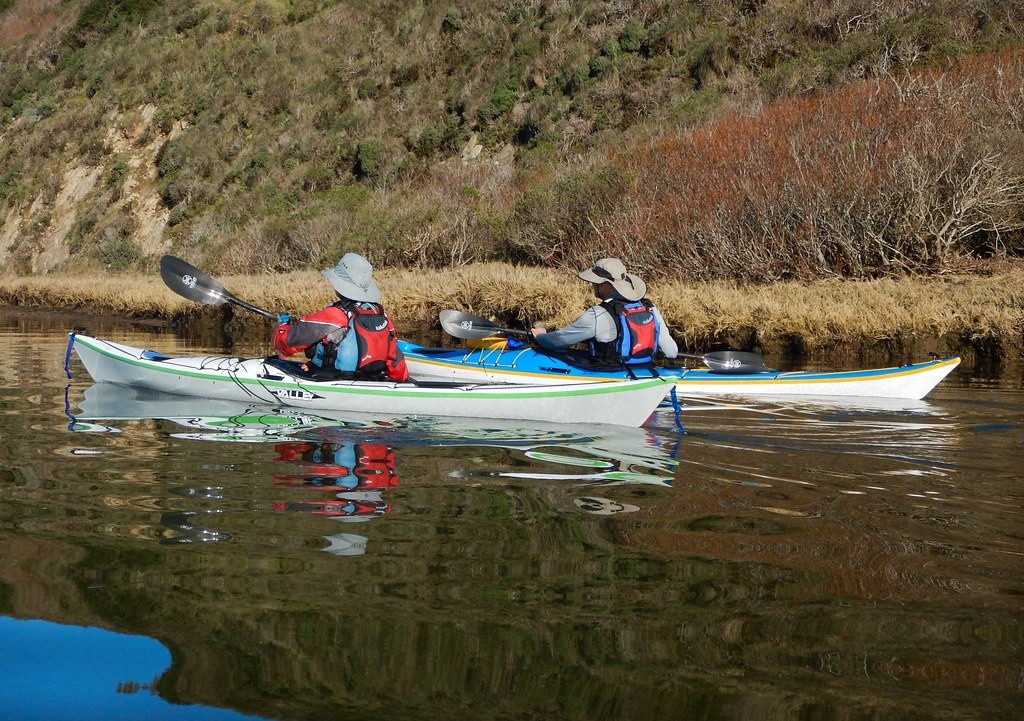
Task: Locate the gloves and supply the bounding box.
[277,311,291,327]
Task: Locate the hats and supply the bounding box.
[322,251,383,302]
[579,258,646,302]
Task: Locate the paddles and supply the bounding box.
[159,254,280,322]
[439,308,765,374]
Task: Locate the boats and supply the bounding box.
[65,331,682,429]
[398,340,961,402]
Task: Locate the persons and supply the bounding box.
[272,252,409,382]
[529,258,679,368]
